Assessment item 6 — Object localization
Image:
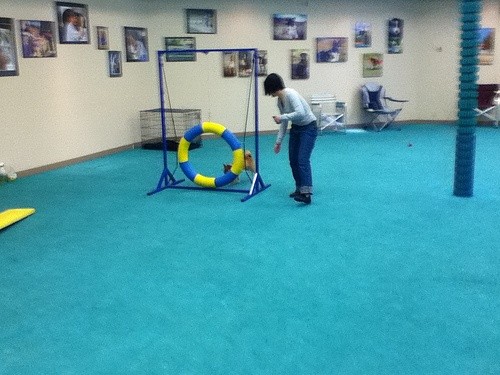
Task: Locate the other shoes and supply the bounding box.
[289,191,300,197]
[294,193,311,203]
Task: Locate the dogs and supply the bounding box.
[284,17,299,40]
[316,39,344,63]
[369,57,384,70]
[388,18,403,40]
[296,53,309,79]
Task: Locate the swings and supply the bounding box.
[161,50,253,188]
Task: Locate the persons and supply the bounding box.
[112,54,119,73]
[264,73,318,204]
[64,11,87,41]
[128,31,148,60]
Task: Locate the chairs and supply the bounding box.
[476,84,500,129]
[362,85,411,132]
[310,95,347,135]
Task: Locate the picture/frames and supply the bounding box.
[0,17,20,77]
[54,1,90,44]
[97,26,110,50]
[165,37,197,61]
[18,19,57,58]
[123,26,149,62]
[185,8,217,34]
[108,51,122,77]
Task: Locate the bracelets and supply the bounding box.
[275,143,281,146]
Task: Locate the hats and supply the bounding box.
[63,9,79,24]
